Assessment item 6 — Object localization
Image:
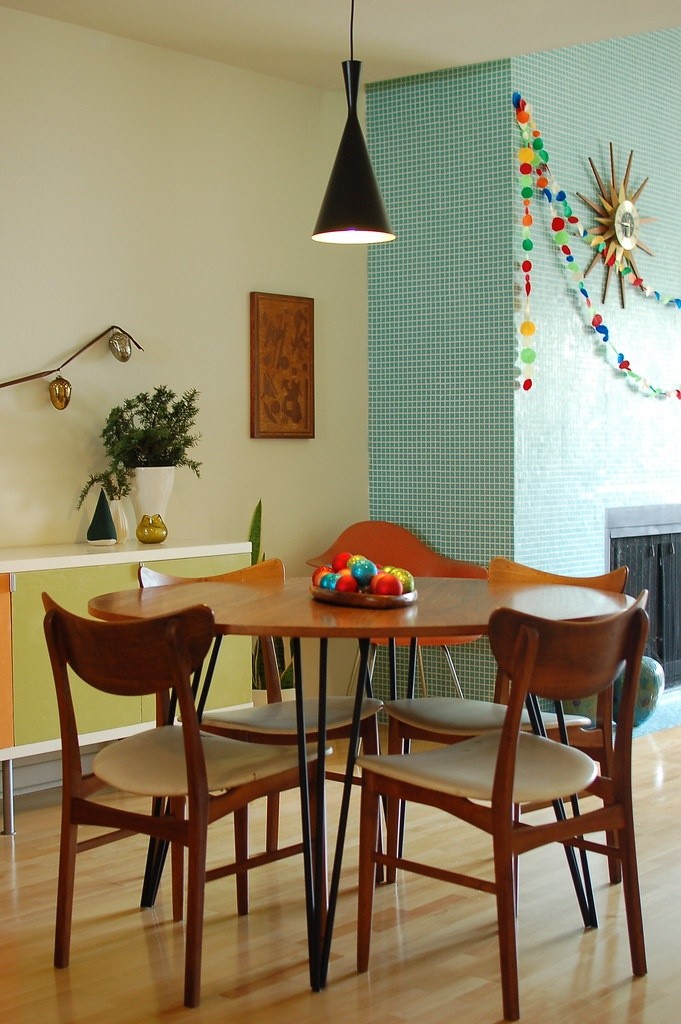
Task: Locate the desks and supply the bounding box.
[88,577,638,973]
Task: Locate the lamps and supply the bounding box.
[310,0,395,245]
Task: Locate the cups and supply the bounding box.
[136,514,167,543]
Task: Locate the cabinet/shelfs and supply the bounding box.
[0,542,253,836]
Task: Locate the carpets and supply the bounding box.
[612,686,681,742]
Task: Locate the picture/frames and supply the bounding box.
[250,292,315,438]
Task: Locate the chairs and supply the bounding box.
[137,521,629,923]
[354,589,651,1022]
[40,591,334,1009]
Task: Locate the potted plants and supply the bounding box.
[98,384,204,526]
[246,499,296,707]
[75,461,137,544]
